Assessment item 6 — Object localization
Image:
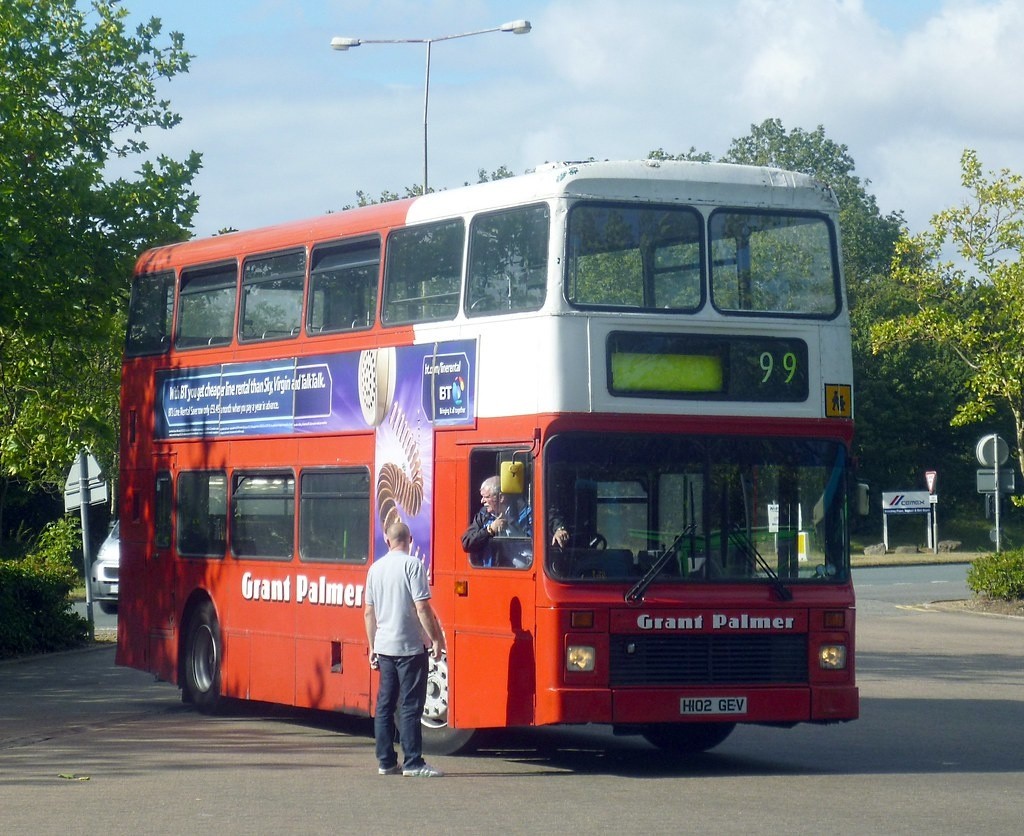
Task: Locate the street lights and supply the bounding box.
[330,20,531,194]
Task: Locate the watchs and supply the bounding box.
[557,526,565,530]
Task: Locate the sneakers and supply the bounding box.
[379,762,403,774]
[403,761,444,777]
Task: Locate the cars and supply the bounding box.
[90,517,122,612]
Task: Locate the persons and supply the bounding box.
[364,522,446,777]
[460,474,570,571]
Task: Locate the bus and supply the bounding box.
[112,158,872,762]
